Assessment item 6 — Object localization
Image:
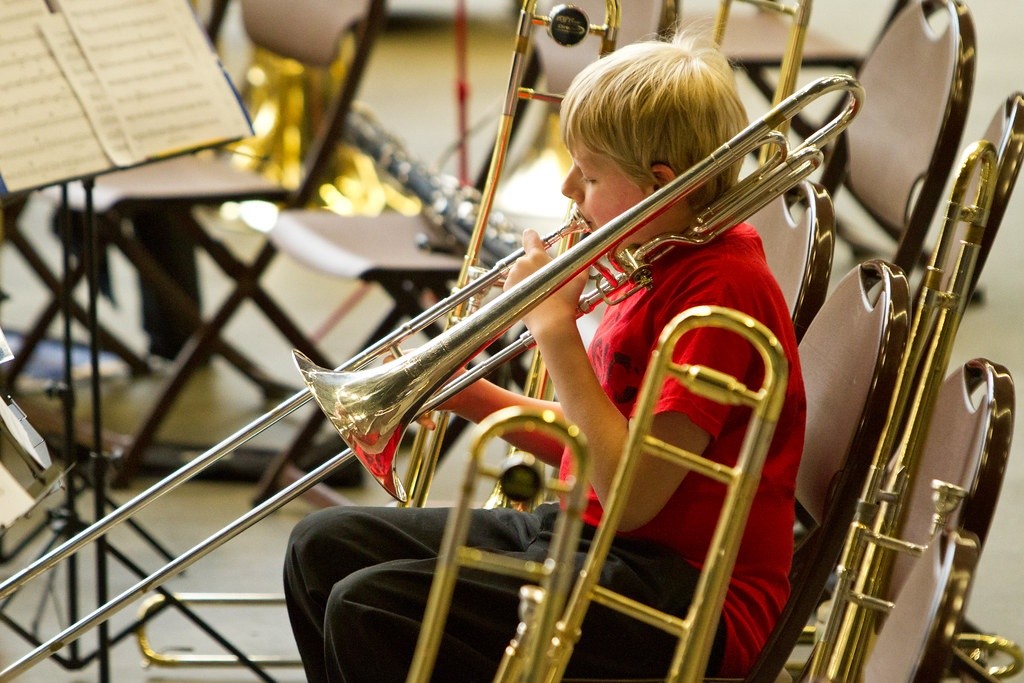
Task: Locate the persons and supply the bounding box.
[54,0,371,373]
[283,21,807,683]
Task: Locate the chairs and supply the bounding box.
[2,0,386,494]
[727,1,979,343]
[750,180,837,343]
[248,0,681,513]
[870,358,1016,641]
[858,524,983,683]
[748,258,925,683]
[746,257,914,683]
[940,91,1024,327]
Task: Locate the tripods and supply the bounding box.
[0,0,277,683]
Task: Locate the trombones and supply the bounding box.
[0,0,1022,681]
